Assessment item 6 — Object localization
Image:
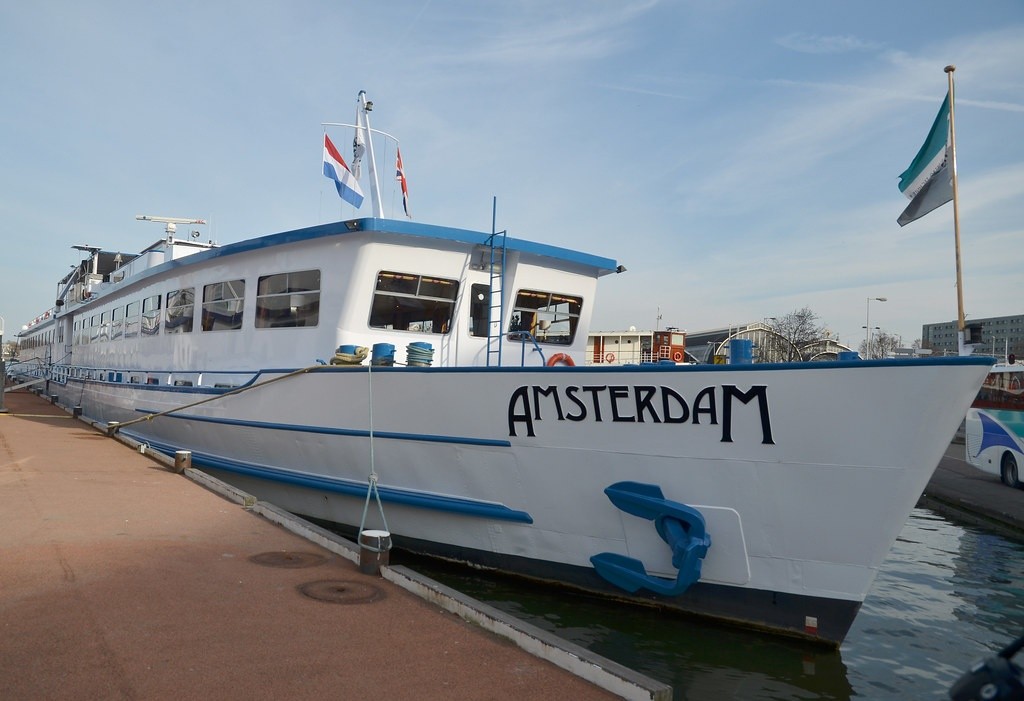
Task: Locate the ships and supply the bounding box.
[5,89,1001,655]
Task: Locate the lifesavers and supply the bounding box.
[605,353,615,362]
[548,352,576,367]
[673,352,683,362]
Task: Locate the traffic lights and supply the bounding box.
[963,324,982,345]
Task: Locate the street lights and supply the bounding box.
[763,317,776,363]
[992,336,995,358]
[866,297,888,362]
[861,326,881,359]
[787,314,805,362]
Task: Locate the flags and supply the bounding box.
[322,134,363,208]
[396,147,408,215]
[895,83,957,227]
[351,106,365,178]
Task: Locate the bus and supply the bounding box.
[965,363,1024,490]
